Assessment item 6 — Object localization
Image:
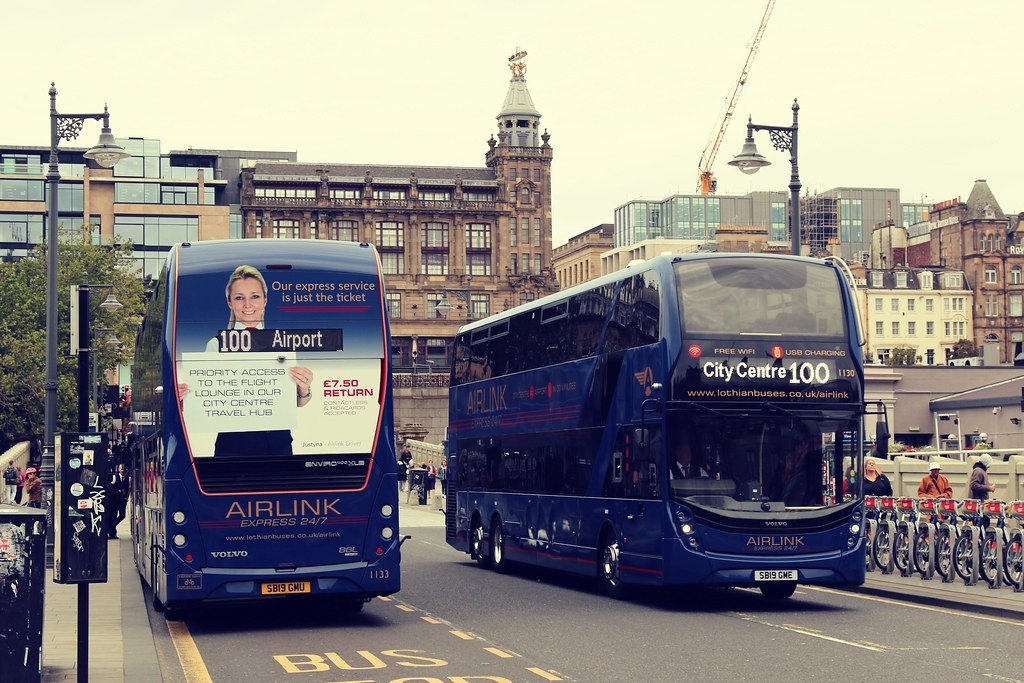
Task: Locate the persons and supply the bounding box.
[968,454,996,528]
[854,460,894,519]
[175,265,313,411]
[438,460,446,498]
[973,432,996,456]
[3,460,42,509]
[918,462,954,523]
[144,459,159,494]
[397,444,437,504]
[841,467,857,498]
[108,441,133,539]
[669,440,711,481]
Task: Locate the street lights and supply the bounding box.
[92,317,121,413]
[729,98,803,254]
[69,268,124,435]
[37,83,133,566]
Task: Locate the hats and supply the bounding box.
[25,468,37,480]
[928,462,942,471]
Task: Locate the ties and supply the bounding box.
[681,466,690,478]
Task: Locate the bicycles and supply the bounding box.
[864,497,1024,587]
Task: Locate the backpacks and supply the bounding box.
[6,469,16,481]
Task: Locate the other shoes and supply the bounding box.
[108,535,119,539]
[442,494,446,498]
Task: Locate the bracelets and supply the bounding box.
[297,387,311,399]
[26,483,29,486]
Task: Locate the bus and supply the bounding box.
[128,238,412,617]
[441,251,892,601]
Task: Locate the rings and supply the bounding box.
[305,379,309,384]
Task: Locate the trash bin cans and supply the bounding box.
[407,469,429,505]
[0,503,49,683]
